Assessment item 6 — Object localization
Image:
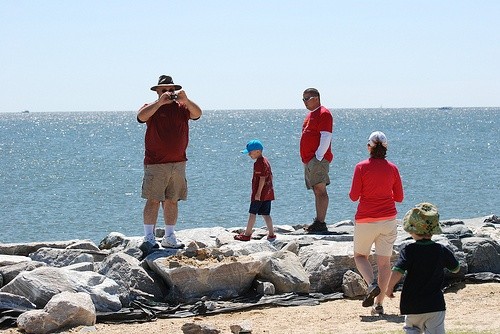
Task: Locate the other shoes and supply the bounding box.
[266,235,277,240]
[234,233,251,241]
[305,222,327,234]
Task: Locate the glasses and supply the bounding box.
[159,88,175,93]
[303,95,318,101]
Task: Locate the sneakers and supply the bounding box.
[144,236,156,247]
[162,233,186,248]
[362,283,381,307]
[371,304,384,316]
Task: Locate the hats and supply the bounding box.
[150,75,182,91]
[367,131,387,148]
[240,141,263,154]
[403,202,444,235]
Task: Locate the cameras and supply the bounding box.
[170,94,178,99]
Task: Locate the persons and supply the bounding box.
[347,131,403,313]
[299,88,333,234]
[234,139,276,241]
[385,202,460,334]
[136,74,202,250]
